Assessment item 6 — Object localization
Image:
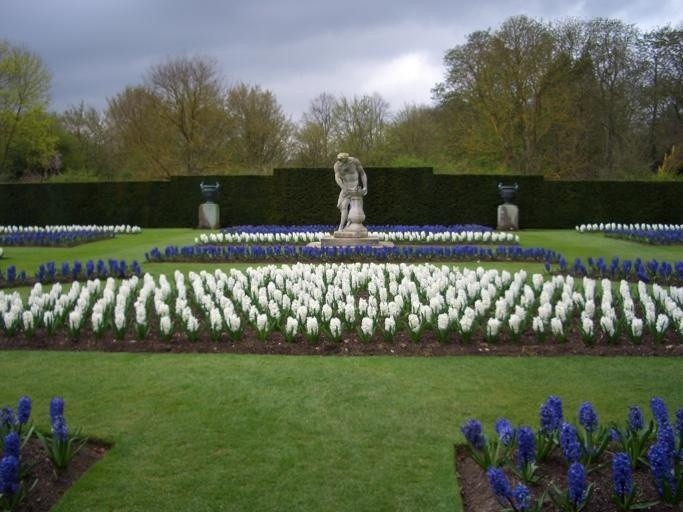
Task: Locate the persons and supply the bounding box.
[333,152,368,232]
